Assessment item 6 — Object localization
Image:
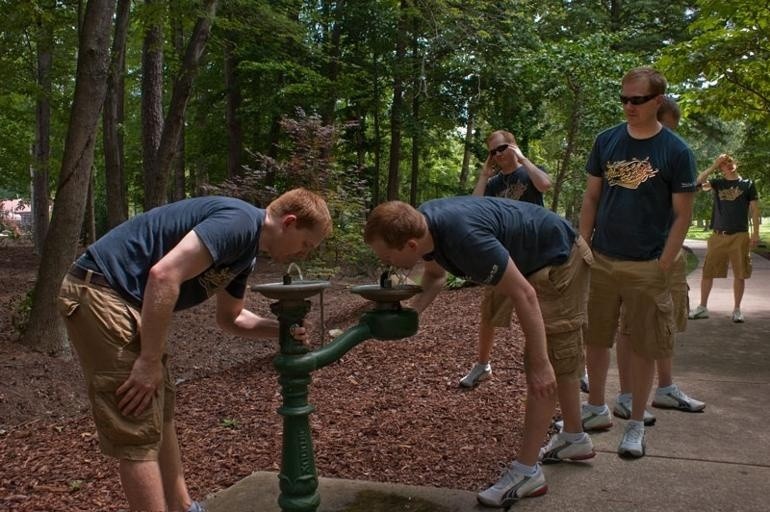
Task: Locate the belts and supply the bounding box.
[67,260,112,285]
[712,229,747,235]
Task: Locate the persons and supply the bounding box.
[57,188,333,511]
[691,153,760,322]
[365,199,596,508]
[554,69,699,459]
[612,97,706,424]
[459,130,591,393]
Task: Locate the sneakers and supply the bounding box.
[580,366,590,393]
[556,404,612,429]
[618,421,644,456]
[479,461,547,505]
[687,306,710,318]
[539,434,595,459]
[652,386,704,410]
[732,308,743,322]
[459,362,492,386]
[614,394,655,423]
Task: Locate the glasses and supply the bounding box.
[490,144,507,155]
[619,96,657,105]
